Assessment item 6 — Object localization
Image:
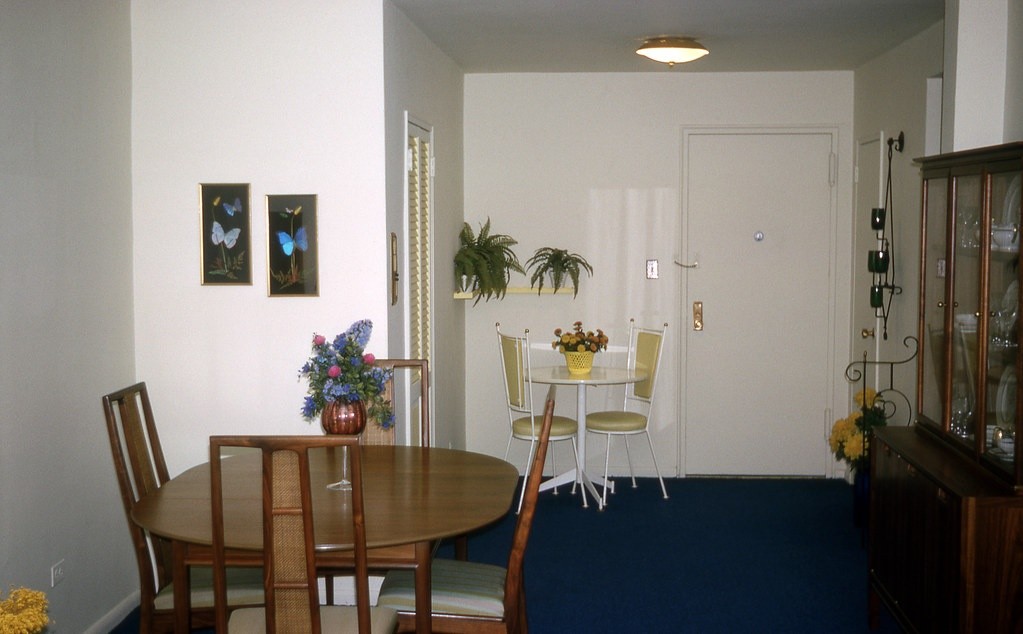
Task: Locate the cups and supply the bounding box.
[998,438,1014,454]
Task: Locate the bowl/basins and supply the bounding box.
[957,313,978,332]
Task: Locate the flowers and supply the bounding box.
[552,321,609,353]
[301,319,396,431]
[829,389,885,464]
[0,587,50,634]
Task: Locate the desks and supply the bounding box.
[128,446,520,634]
[526,367,646,510]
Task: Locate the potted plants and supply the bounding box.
[525,246,593,301]
[453,216,525,308]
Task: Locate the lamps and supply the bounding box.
[867,130,903,342]
[635,36,709,68]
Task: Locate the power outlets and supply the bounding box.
[51,559,65,587]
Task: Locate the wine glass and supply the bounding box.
[990,292,1019,347]
[950,391,975,438]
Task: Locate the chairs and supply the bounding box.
[102,381,265,634]
[320,360,432,634]
[376,384,557,634]
[497,322,588,515]
[210,435,398,634]
[587,318,669,506]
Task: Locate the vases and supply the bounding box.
[322,397,366,436]
[563,350,595,375]
[852,456,871,533]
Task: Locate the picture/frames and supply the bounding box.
[197,182,252,286]
[265,194,318,298]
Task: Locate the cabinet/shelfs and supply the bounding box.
[865,138,1023,634]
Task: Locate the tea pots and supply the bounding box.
[993,223,1020,250]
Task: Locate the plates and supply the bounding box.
[995,362,1017,430]
[988,447,1015,459]
[1001,174,1020,226]
[1002,279,1019,330]
